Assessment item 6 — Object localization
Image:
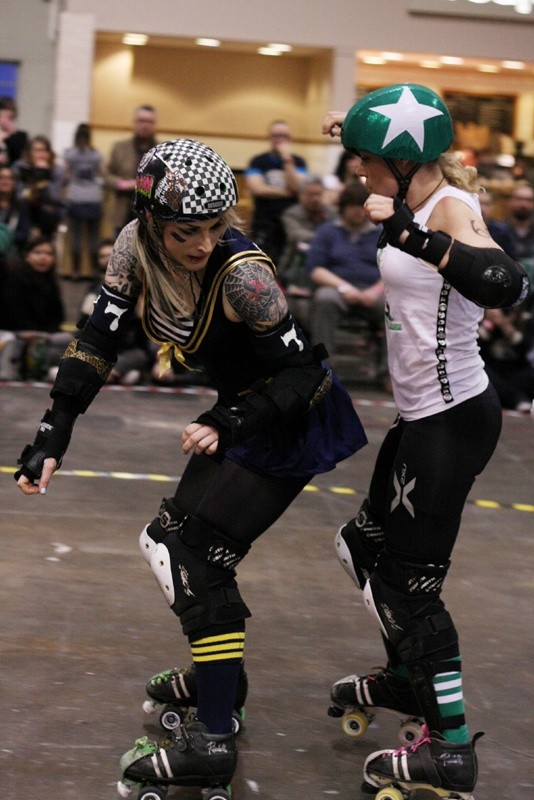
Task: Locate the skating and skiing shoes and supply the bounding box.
[327,657,428,749]
[117,722,237,800]
[142,660,247,735]
[360,725,486,800]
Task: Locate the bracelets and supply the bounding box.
[338,284,348,293]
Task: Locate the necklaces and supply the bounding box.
[411,176,445,211]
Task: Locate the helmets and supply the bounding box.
[341,84,455,163]
[134,138,240,221]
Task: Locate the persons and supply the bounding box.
[276,150,534,414]
[62,124,108,279]
[0,97,65,334]
[107,105,159,235]
[14,138,370,800]
[321,84,533,800]
[245,122,308,260]
